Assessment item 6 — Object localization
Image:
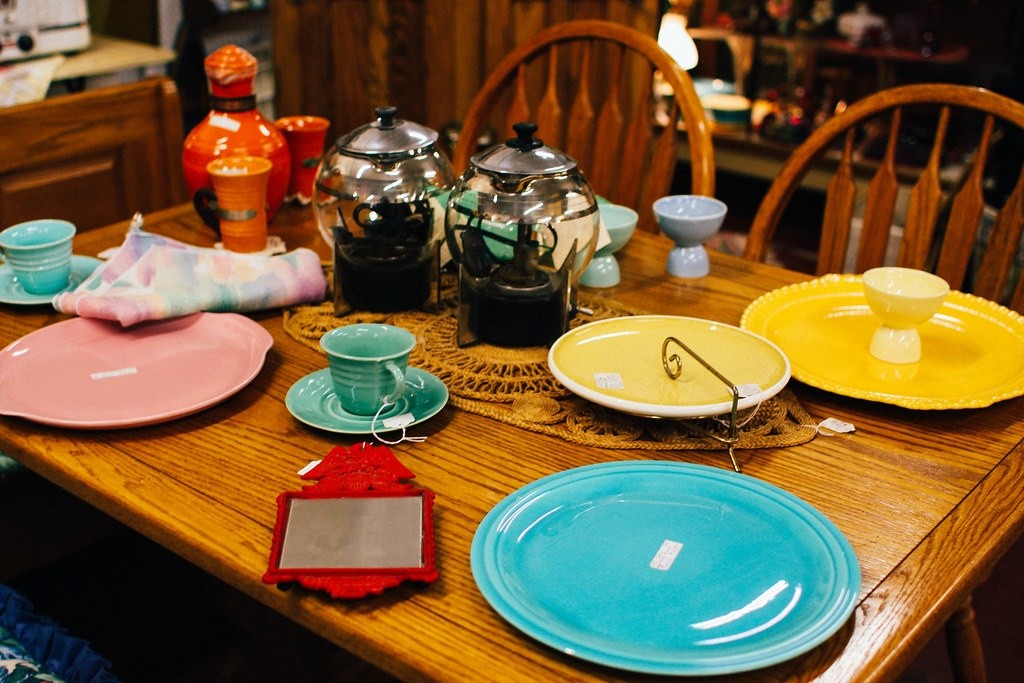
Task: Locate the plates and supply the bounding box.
[286,366,449,433]
[739,273,1024,411]
[0,255,105,305]
[1,313,274,430]
[470,459,862,676]
[547,314,792,421]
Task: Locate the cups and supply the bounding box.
[274,115,331,202]
[194,155,273,252]
[320,323,417,415]
[0,219,77,293]
[654,75,753,135]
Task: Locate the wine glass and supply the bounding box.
[577,195,729,289]
[864,266,951,364]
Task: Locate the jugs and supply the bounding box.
[312,105,602,348]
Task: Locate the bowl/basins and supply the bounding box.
[460,190,610,263]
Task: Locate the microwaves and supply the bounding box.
[1,0,92,65]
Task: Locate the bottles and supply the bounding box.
[181,43,291,222]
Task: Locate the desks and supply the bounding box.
[0,32,178,109]
[648,91,979,278]
[0,196,1024,683]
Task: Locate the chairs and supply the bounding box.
[0,76,192,237]
[453,18,717,239]
[742,84,1024,683]
